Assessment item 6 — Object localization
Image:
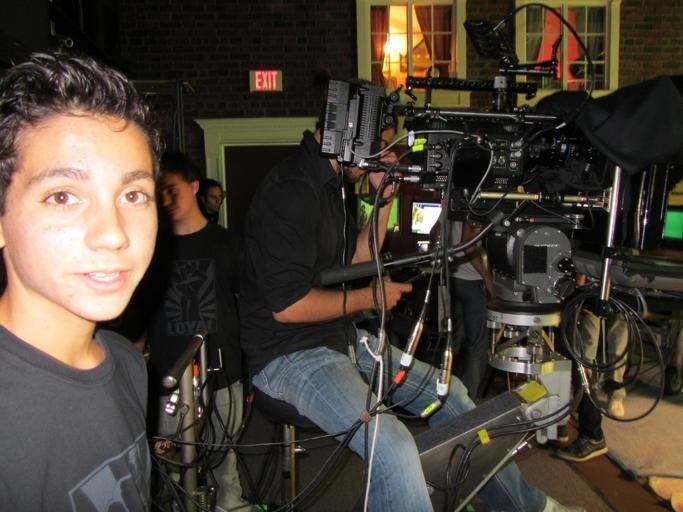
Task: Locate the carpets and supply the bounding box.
[601,393,683,479]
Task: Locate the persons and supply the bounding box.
[92,79,635,511]
[0,52,167,512]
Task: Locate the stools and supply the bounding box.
[254,389,316,506]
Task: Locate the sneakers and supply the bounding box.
[556,436,608,461]
[608,399,624,416]
